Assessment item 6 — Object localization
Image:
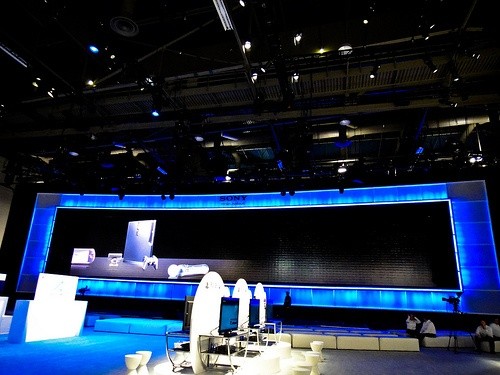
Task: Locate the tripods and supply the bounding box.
[448,310,479,354]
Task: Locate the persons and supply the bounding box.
[416,315,436,345]
[406,314,421,337]
[474,319,500,354]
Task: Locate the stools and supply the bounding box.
[305,351,322,374]
[125,351,152,375]
[313,340,324,360]
[306,354,320,375]
[310,342,323,362]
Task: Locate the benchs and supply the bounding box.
[84,315,184,336]
[262,325,500,352]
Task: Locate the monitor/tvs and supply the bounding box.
[249,299,260,329]
[182,296,194,330]
[219,297,238,337]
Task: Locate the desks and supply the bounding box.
[198,322,282,375]
[165,331,190,373]
